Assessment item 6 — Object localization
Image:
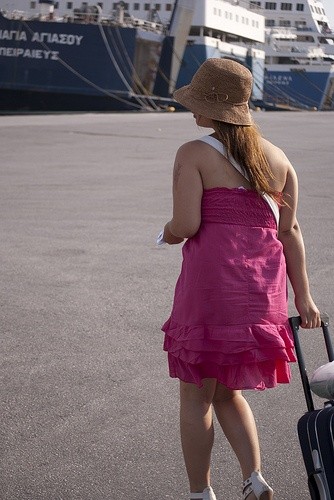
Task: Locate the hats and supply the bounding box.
[172,58,255,126]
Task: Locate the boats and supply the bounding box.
[2,0,264,112]
[254,2,334,112]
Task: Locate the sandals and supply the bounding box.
[241,471,274,500]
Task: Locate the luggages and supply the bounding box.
[287,312,334,500]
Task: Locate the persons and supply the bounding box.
[155,58,322,500]
